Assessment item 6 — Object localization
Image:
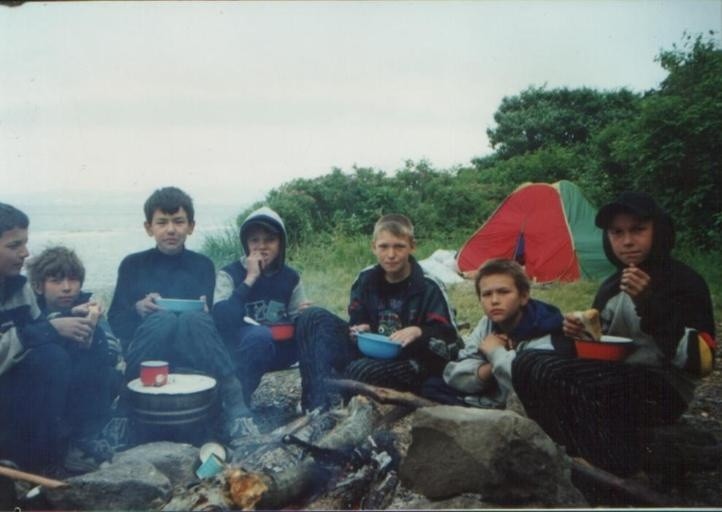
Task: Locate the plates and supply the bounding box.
[149,296,205,315]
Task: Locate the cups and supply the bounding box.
[141,361,170,387]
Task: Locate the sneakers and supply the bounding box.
[229,417,260,440]
[65,416,128,473]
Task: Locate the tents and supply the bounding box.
[459,179,618,289]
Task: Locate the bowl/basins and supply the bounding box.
[261,321,297,341]
[354,331,404,360]
[572,334,633,360]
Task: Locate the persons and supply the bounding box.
[340,213,465,424]
[419,260,563,410]
[563,191,714,468]
[0,205,93,494]
[91,191,263,454]
[211,212,346,414]
[29,248,119,473]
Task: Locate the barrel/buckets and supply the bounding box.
[125,374,219,447]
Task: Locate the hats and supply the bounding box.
[595,190,655,230]
[244,221,280,235]
